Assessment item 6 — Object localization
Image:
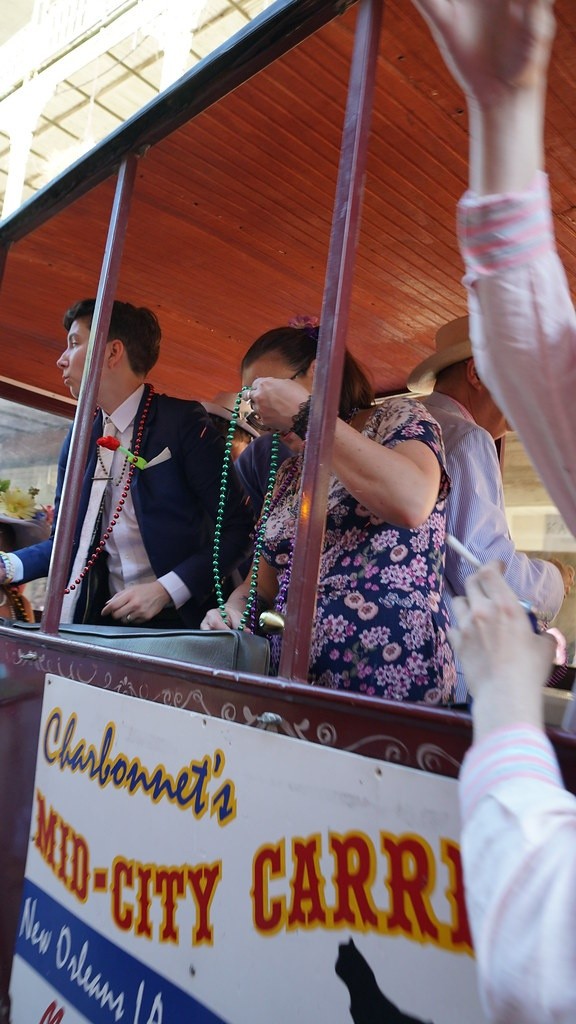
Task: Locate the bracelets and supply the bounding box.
[0,551,14,583]
[292,395,339,441]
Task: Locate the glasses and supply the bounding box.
[245,366,306,432]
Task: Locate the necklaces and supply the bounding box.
[251,458,303,638]
[64,382,154,594]
[214,385,279,630]
[0,589,29,622]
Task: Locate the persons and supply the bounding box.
[406,315,574,702]
[0,299,255,630]
[200,316,457,709]
[410,0,576,1024]
[200,391,296,608]
[0,514,51,624]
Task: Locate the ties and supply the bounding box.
[58,420,119,624]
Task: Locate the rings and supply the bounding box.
[247,399,255,410]
[248,390,251,398]
[127,614,133,622]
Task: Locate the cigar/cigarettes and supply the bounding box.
[445,534,482,569]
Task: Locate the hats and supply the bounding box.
[406,315,473,394]
